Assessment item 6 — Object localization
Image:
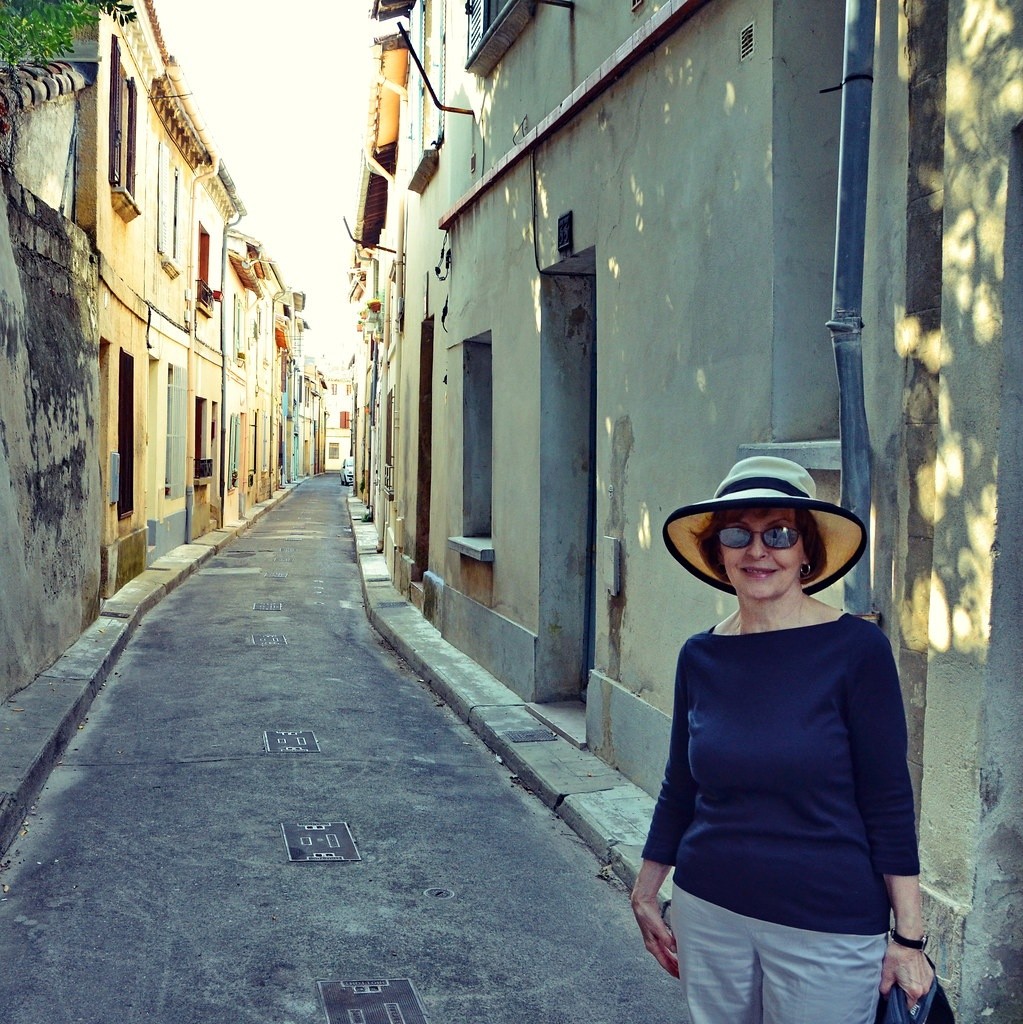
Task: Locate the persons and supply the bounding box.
[631,455,927,1024]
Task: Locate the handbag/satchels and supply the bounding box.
[887,972,955,1024]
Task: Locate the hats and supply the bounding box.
[663,456,868,596]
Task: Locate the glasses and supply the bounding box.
[716,526,802,549]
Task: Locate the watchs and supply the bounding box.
[891,928,928,952]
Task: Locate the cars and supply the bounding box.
[339,457,354,486]
